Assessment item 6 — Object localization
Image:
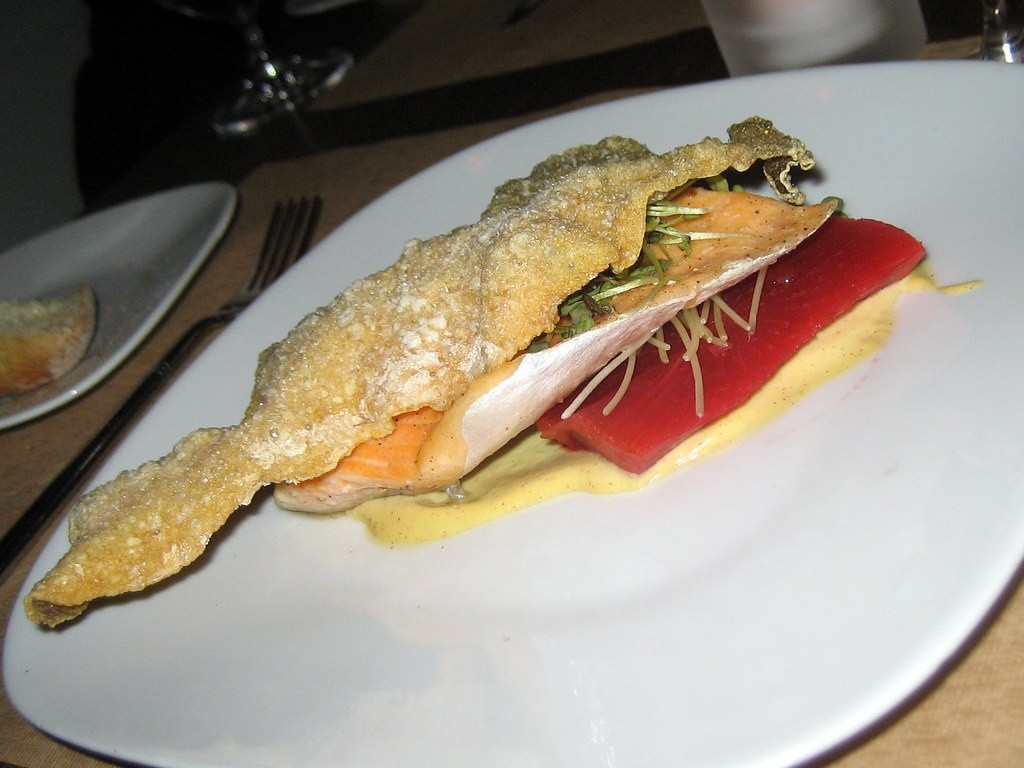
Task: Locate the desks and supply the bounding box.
[0,36,1024,767]
[308,0,709,110]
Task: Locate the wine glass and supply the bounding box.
[177,1,354,136]
[964,0,1024,63]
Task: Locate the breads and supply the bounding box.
[0,288,98,401]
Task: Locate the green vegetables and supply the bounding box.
[523,174,848,422]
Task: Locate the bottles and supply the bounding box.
[701,1,928,83]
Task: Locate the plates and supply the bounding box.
[1,180,238,431]
[0,57,1024,766]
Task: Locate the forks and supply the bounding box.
[1,193,324,581]
